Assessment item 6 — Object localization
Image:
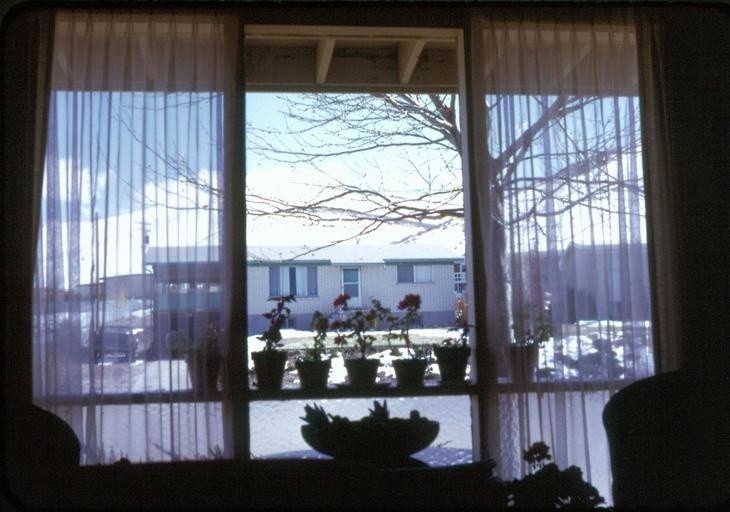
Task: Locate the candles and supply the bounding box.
[251,290,297,390]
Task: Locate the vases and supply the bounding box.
[432,344,469,386]
[295,361,330,389]
[392,359,427,389]
[344,358,379,385]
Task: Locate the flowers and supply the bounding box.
[369,292,431,359]
[296,293,352,361]
[331,306,381,359]
[436,296,469,348]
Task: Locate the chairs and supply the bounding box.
[602,368,730,512]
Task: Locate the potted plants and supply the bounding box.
[505,298,552,383]
[184,324,221,391]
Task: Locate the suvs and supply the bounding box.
[87,315,151,364]
